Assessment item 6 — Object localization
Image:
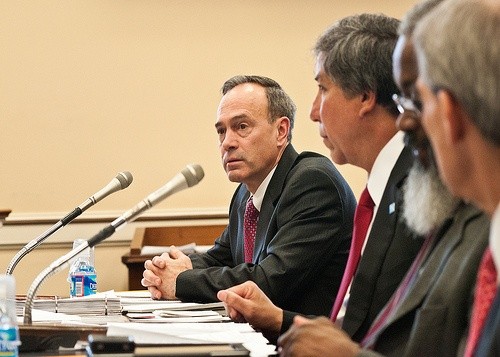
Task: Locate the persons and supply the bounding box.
[277,0,499,357]
[140,73,358,318]
[215,10,427,328]
[410,1,500,357]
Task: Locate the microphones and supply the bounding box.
[7,171,132,275]
[16,165,205,352]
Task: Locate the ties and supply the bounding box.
[462,245,496,355]
[359,229,434,350]
[244,196,260,264]
[330,187,375,323]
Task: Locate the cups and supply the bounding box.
[69,239,95,283]
[0,275,18,345]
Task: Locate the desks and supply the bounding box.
[122,225,228,290]
[0,295,288,357]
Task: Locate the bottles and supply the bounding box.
[0,318,19,357]
[69,257,97,299]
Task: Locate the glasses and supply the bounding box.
[391,93,421,118]
